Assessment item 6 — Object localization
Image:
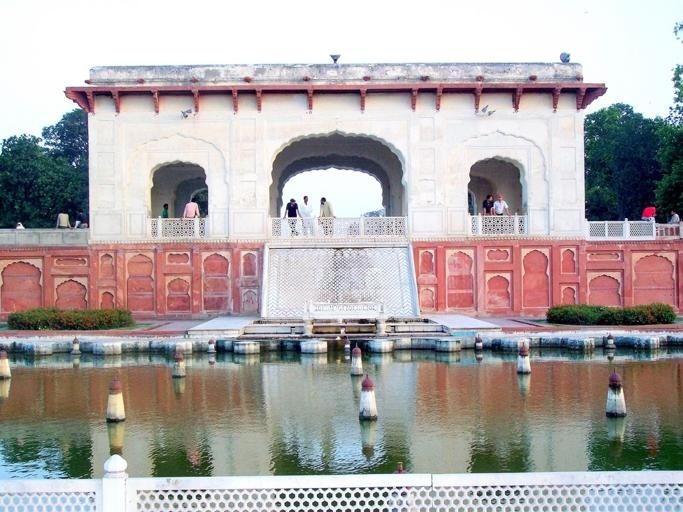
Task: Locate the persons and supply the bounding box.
[16,222,25,229]
[283,196,314,235]
[318,197,336,236]
[183,198,200,218]
[667,209,680,224]
[73,209,88,228]
[483,194,512,215]
[161,203,168,218]
[56,208,73,228]
[640,203,657,221]
